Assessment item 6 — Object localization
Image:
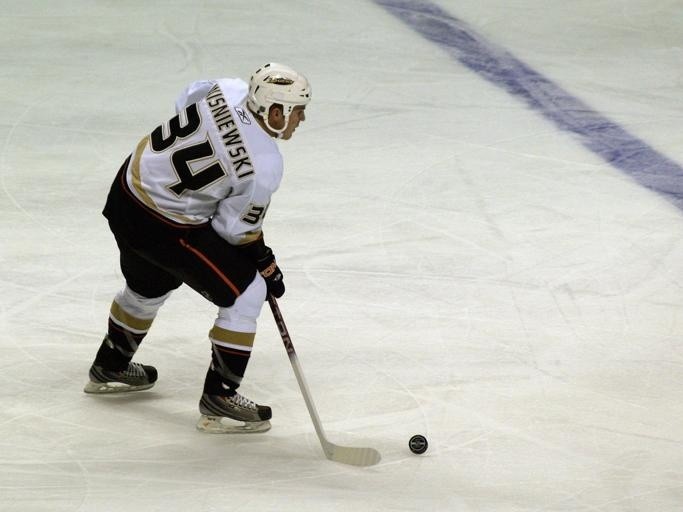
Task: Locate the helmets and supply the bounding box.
[246,62,313,139]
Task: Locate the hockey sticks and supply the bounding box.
[265,294,381,466]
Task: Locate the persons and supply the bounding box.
[87,62,312,421]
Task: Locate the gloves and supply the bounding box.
[252,248,285,298]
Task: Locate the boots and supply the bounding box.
[88,335,160,385]
[200,362,272,423]
